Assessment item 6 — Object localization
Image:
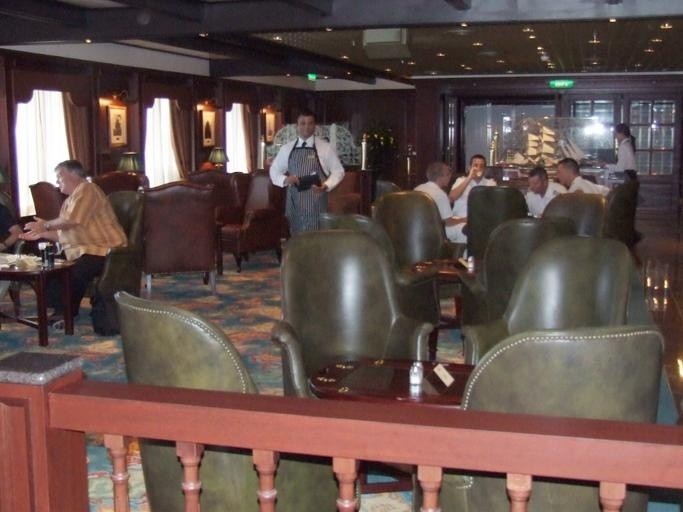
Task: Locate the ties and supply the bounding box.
[301,141,307,149]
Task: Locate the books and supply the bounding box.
[294,172,323,192]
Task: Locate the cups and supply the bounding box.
[40,244,54,269]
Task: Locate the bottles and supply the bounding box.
[410,361,423,385]
[466,256,473,274]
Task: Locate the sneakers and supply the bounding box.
[49,311,81,325]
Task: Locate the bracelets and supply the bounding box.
[44,221,51,231]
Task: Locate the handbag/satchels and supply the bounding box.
[91,287,140,335]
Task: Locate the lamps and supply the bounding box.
[200,109,216,147]
[117,151,139,172]
[207,146,229,170]
[107,105,129,148]
[264,112,275,143]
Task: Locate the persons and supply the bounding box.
[0,202,22,296]
[553,156,609,203]
[597,123,646,246]
[16,159,126,328]
[411,160,469,263]
[519,165,563,219]
[268,107,344,234]
[446,152,496,216]
[113,114,122,135]
